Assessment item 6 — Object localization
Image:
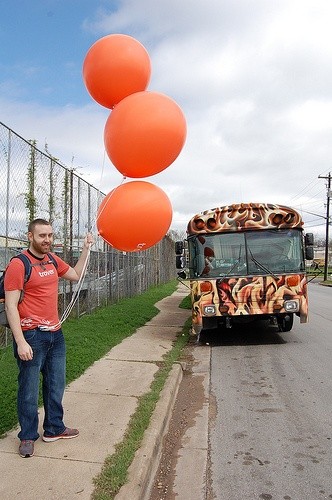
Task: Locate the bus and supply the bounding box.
[174,203,314,341]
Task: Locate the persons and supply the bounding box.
[3,218,92,458]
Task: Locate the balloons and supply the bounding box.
[104,91,188,179]
[82,33,151,109]
[97,180,173,253]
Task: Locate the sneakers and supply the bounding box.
[42,427,79,442]
[19,440,34,458]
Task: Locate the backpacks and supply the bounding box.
[0,253,57,329]
